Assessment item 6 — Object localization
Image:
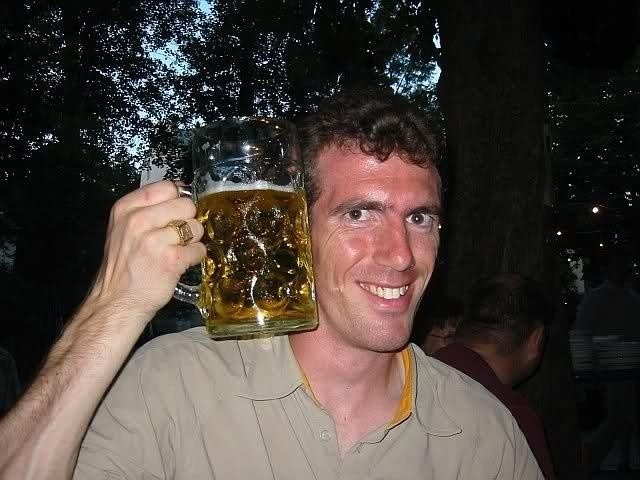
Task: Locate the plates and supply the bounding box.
[570,329,640,372]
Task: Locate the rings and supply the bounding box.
[165,220,194,246]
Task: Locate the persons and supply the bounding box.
[430,271,559,480]
[410,297,467,357]
[0,88,545,480]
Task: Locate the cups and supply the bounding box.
[172,119,318,341]
